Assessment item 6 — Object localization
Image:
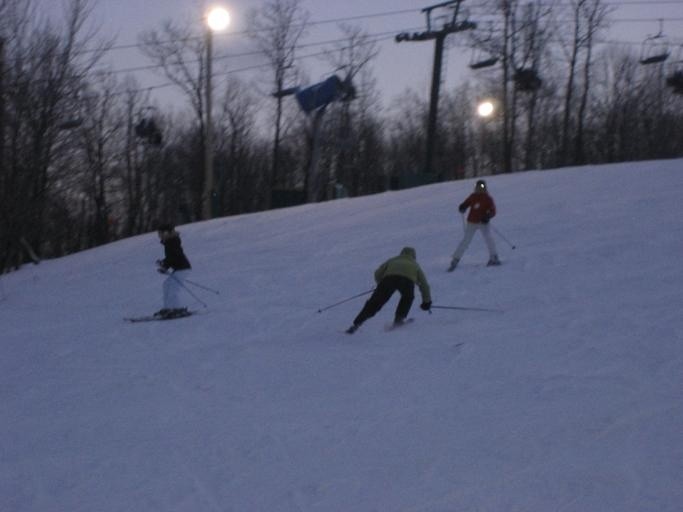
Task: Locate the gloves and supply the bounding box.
[420,300,433,311]
[481,216,489,223]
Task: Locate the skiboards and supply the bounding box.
[326,318,414,334]
[123,305,197,323]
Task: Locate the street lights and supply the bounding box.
[202,7,229,221]
[477,101,495,175]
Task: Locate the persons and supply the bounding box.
[153,221,193,318]
[443,178,505,275]
[340,244,433,336]
[0,199,121,274]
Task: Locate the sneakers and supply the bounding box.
[349,317,404,332]
[449,258,501,268]
